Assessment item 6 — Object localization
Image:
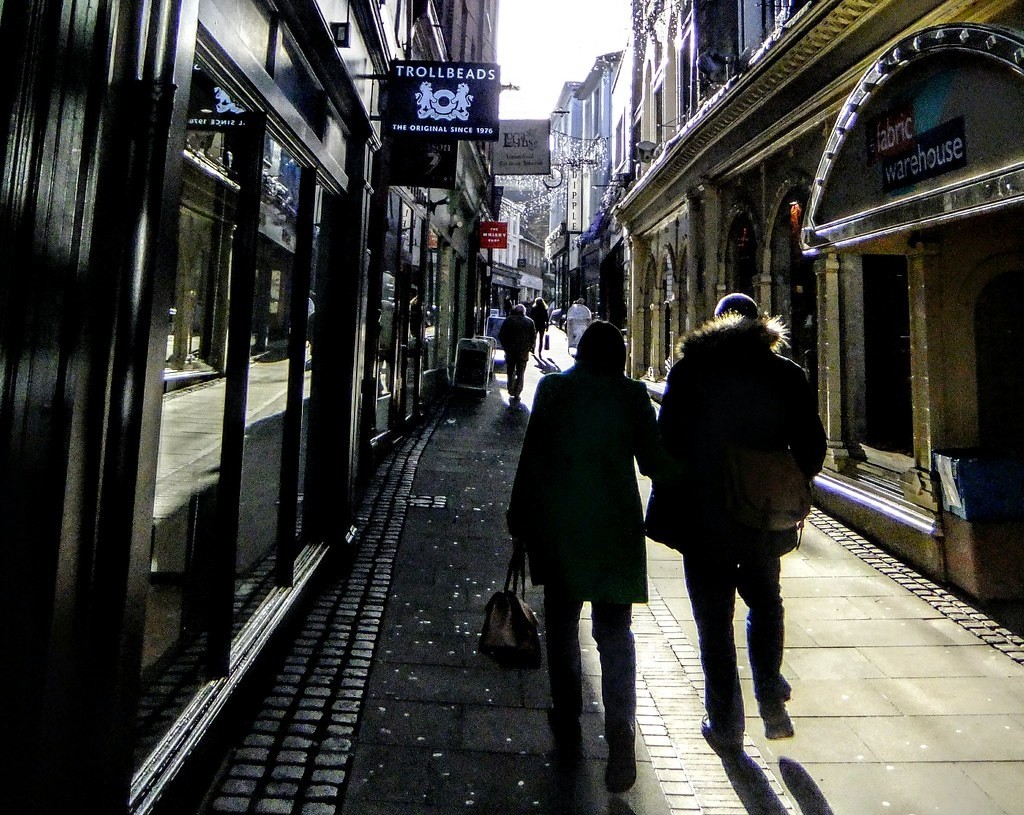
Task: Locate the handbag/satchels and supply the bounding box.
[723,445,811,531]
[545,331,549,350]
[478,549,543,670]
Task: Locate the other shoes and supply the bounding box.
[548,703,582,745]
[759,701,793,740]
[605,722,636,793]
[701,714,744,758]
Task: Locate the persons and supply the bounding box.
[499,297,549,399]
[645,293,827,752]
[506,320,668,792]
[558,298,585,330]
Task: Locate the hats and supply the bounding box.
[715,293,758,319]
